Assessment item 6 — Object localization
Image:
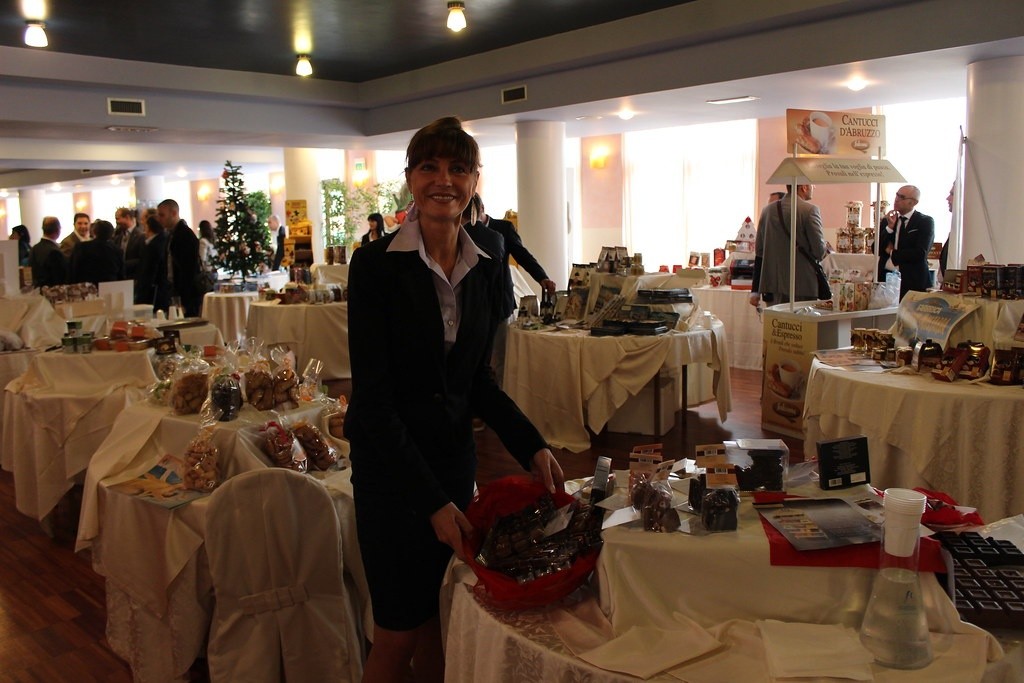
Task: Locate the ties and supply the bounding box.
[898,216,907,251]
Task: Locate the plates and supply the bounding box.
[768,382,803,403]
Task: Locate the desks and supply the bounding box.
[801,338,1024,528]
[203,292,259,346]
[500,291,733,451]
[1,353,161,521]
[74,357,377,683]
[97,317,224,351]
[436,465,1023,683]
[249,303,351,379]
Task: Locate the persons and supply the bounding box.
[266,216,285,270]
[199,220,219,274]
[60,199,206,317]
[460,192,556,431]
[749,185,827,307]
[31,218,68,288]
[11,226,28,263]
[871,186,934,304]
[361,213,390,248]
[344,118,565,683]
[937,181,954,288]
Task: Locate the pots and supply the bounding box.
[773,401,800,424]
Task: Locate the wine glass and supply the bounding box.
[756,294,767,323]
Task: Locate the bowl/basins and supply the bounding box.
[459,476,603,610]
[132,305,154,321]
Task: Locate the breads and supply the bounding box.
[485,498,589,577]
[145,367,349,491]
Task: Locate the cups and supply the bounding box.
[882,488,926,557]
[779,358,801,388]
[673,265,682,272]
[157,310,164,322]
[714,249,725,266]
[168,307,177,321]
[660,266,668,272]
[687,252,710,267]
[725,240,750,252]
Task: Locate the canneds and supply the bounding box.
[307,288,331,304]
[609,253,644,277]
[944,273,962,290]
[850,327,913,367]
[61,320,92,354]
[213,283,218,293]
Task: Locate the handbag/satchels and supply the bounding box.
[193,271,213,295]
[815,262,832,300]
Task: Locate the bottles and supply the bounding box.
[630,252,644,275]
[540,287,554,324]
[609,256,626,277]
[873,348,896,361]
[836,201,890,254]
[704,315,711,329]
[945,273,961,291]
[171,295,185,320]
[858,525,934,669]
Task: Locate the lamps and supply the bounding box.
[296,53,312,76]
[446,1,467,33]
[24,20,49,49]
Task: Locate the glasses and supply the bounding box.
[895,193,915,200]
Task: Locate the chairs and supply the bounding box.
[205,468,364,683]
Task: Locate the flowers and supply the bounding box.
[206,160,277,292]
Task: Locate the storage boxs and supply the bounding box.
[739,448,785,492]
[817,436,871,490]
[609,377,677,434]
[135,305,154,319]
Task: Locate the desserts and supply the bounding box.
[630,479,739,532]
